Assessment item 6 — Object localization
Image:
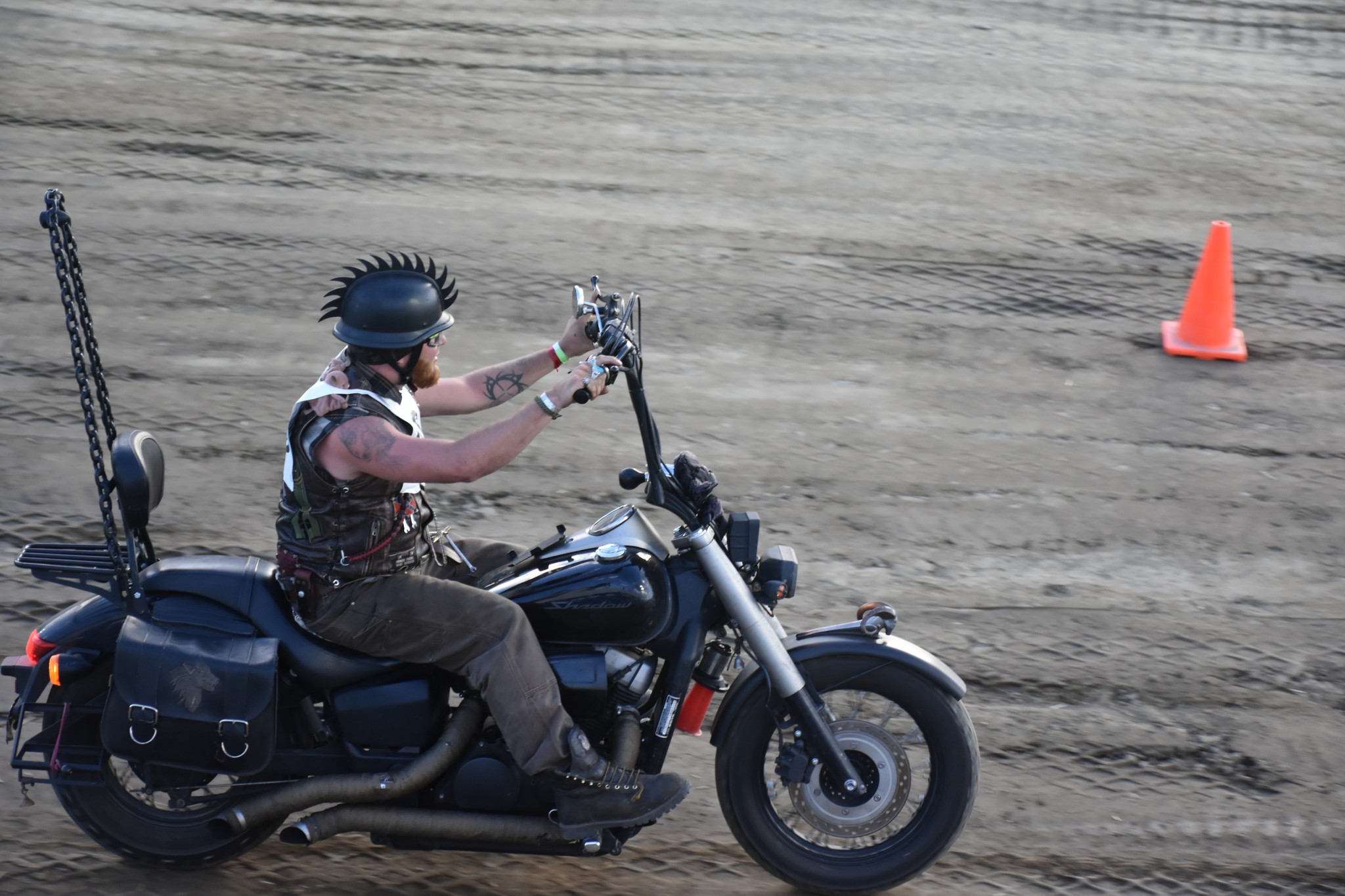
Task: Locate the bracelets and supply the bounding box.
[533,395,563,420]
[552,341,571,364]
[540,391,561,414]
[547,346,563,372]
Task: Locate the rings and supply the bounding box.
[582,377,591,389]
[587,355,598,367]
[590,365,605,380]
[593,341,600,349]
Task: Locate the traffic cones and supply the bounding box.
[1158,218,1251,366]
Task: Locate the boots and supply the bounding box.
[546,723,692,835]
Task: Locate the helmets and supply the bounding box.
[316,250,458,348]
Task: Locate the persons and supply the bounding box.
[275,277,689,838]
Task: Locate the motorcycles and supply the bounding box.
[0,188,982,896]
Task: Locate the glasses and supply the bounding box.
[424,330,442,347]
